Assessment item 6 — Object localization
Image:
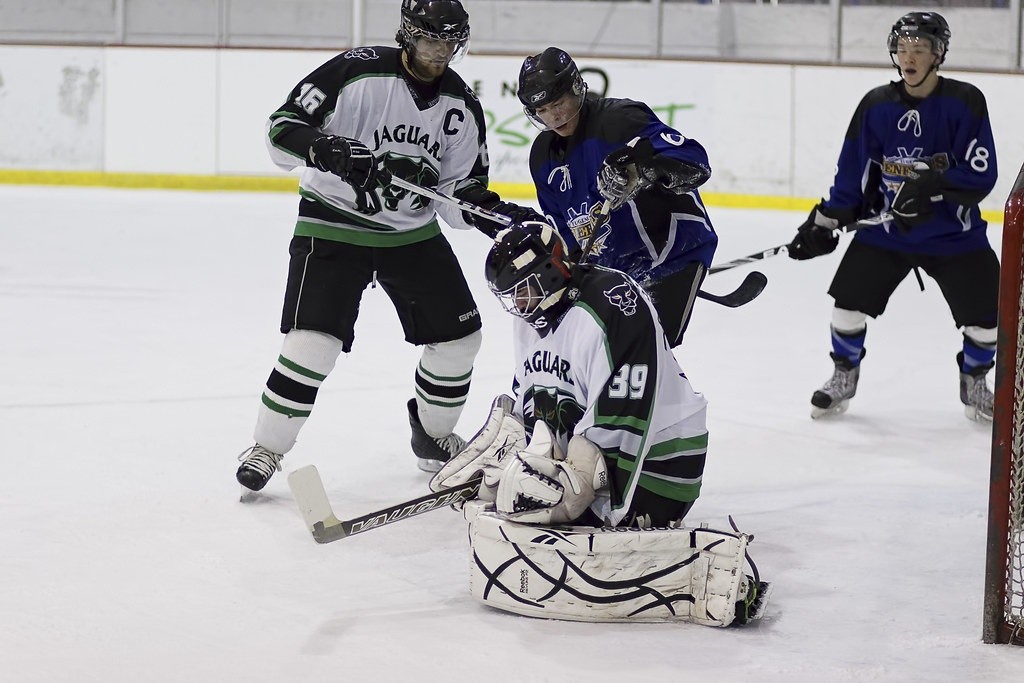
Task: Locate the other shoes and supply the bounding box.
[732,572,771,626]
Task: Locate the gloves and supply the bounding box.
[888,171,961,227]
[595,137,659,212]
[787,203,841,261]
[473,200,551,240]
[312,136,379,192]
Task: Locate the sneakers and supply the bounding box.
[810,347,866,423]
[956,351,995,425]
[236,441,284,502]
[406,397,469,472]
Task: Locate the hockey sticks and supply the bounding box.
[705,192,944,282]
[372,170,769,309]
[582,172,627,269]
[286,462,486,545]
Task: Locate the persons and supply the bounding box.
[788,11,1000,424]
[516,47,719,348]
[236,0,536,502]
[429,221,770,629]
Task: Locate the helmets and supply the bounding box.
[515,46,583,116]
[397,0,469,48]
[484,222,586,338]
[888,11,952,57]
